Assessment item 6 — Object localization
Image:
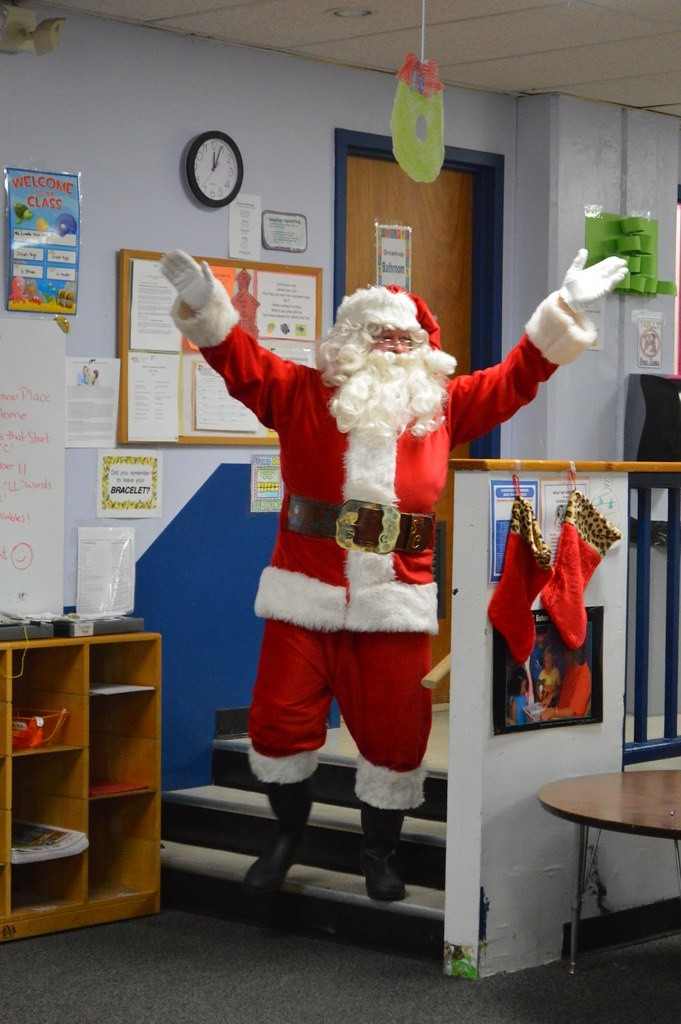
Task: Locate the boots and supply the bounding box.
[360,802,408,901]
[242,783,313,896]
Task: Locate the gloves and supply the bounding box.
[558,248,629,314]
[159,248,218,311]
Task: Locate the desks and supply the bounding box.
[538,770,681,977]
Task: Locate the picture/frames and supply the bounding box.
[493,605,605,735]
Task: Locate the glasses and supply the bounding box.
[374,338,416,349]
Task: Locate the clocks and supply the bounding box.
[184,129,245,208]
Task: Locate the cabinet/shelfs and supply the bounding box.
[0,633,162,943]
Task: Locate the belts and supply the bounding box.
[285,493,435,554]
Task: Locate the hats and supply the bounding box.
[333,284,457,375]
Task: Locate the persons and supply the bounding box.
[506,626,591,725]
[156,247,629,902]
